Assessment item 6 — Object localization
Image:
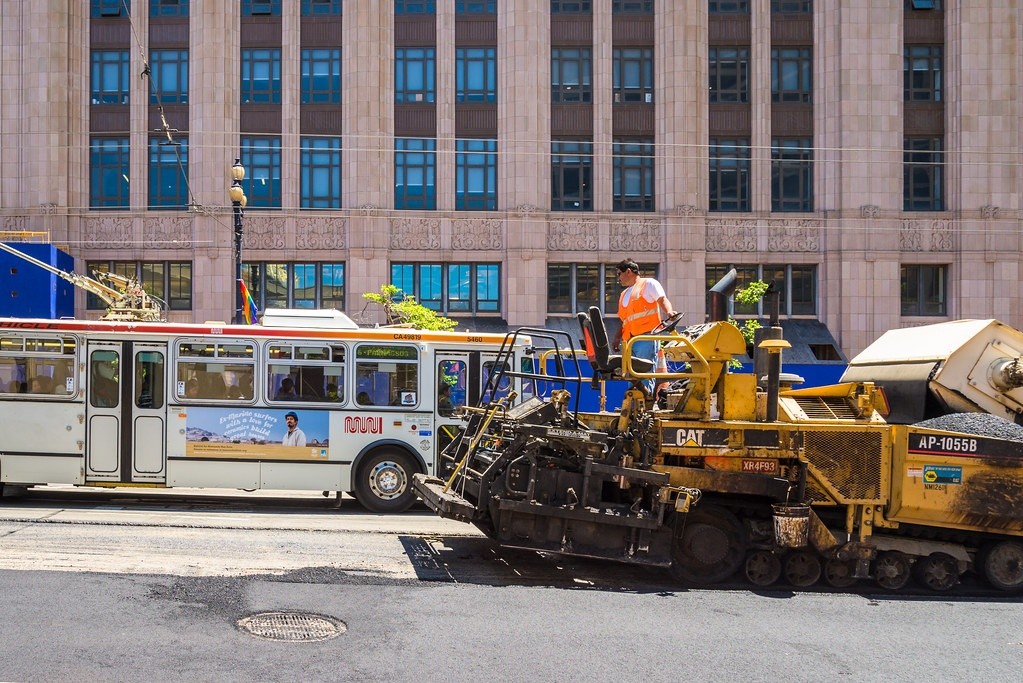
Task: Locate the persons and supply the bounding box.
[9,381,21,392]
[283,411,306,447]
[326,383,340,402]
[276,378,297,400]
[612,259,678,413]
[392,388,410,406]
[185,350,253,398]
[357,392,374,405]
[438,383,454,416]
[30,359,72,395]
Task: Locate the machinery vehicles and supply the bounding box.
[409,269,1023,599]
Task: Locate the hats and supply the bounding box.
[285,411,298,422]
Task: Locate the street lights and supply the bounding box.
[226,156,250,327]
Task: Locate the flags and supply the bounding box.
[241,282,258,324]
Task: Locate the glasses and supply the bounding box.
[617,272,622,277]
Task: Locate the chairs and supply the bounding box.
[577,306,653,391]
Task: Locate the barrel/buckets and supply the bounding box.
[770,486,810,547]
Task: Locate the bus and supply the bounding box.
[0,241,535,516]
